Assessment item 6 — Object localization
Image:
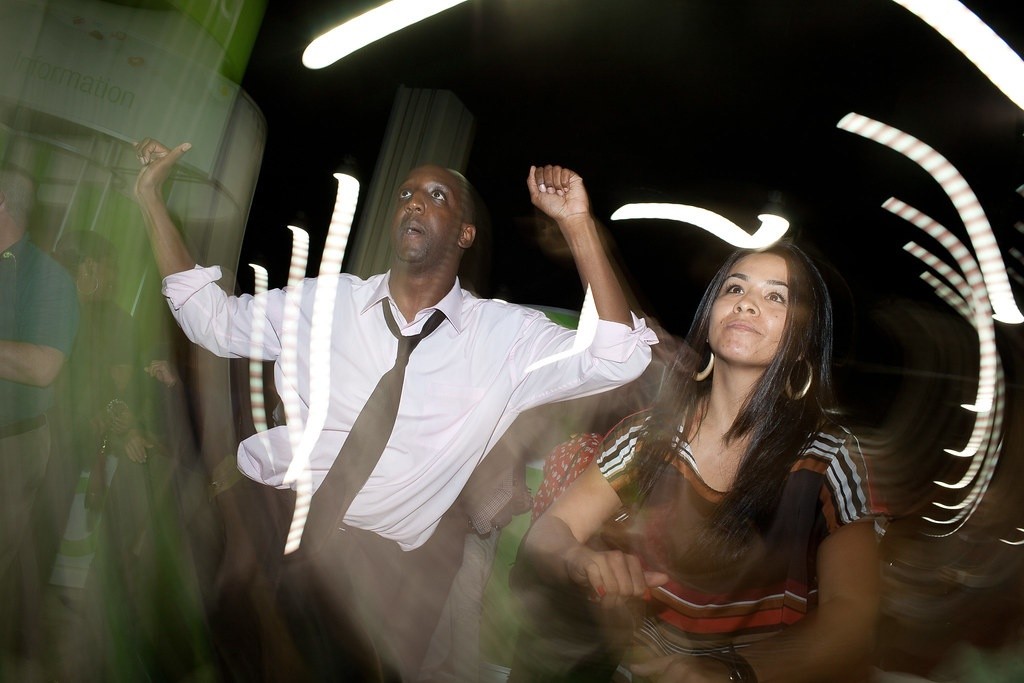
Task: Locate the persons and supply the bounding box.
[0,164,127,660]
[133,137,659,683]
[524,240,888,683]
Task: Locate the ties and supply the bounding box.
[298,298,446,563]
